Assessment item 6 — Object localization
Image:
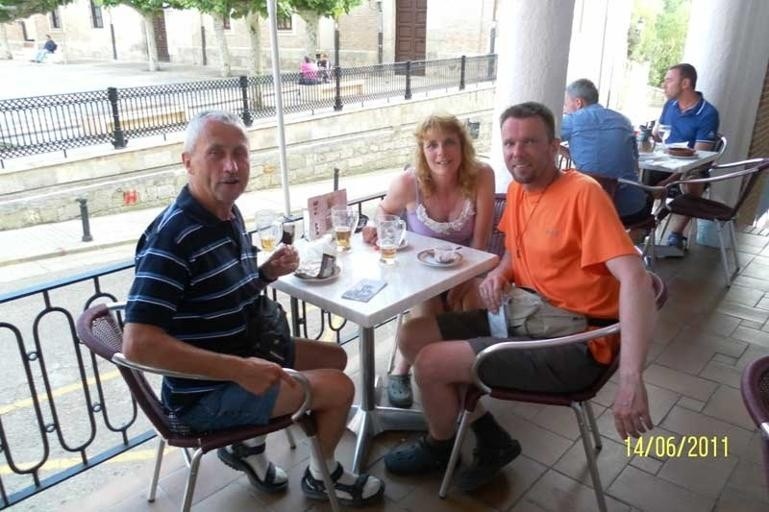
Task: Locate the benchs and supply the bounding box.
[296,78,367,104]
[81,102,191,135]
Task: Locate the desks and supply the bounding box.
[561,141,722,272]
[252,218,508,477]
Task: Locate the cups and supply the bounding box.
[373,212,409,266]
[254,209,286,251]
[436,243,452,263]
[331,203,358,255]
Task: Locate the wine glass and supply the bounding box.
[657,123,673,144]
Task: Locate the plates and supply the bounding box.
[295,263,344,283]
[376,239,407,249]
[418,248,464,269]
[665,147,696,155]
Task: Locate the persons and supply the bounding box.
[384,100,658,493]
[122,106,386,505]
[300,50,331,85]
[558,77,649,230]
[643,62,721,251]
[359,110,497,412]
[31,33,57,65]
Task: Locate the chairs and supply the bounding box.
[385,192,508,378]
[645,132,730,256]
[738,352,769,510]
[640,154,769,292]
[72,298,367,511]
[438,266,670,512]
[560,165,669,266]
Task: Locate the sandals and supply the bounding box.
[302,461,384,504]
[218,442,288,492]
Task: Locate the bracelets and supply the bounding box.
[258,269,278,284]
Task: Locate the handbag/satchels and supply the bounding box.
[504,288,587,338]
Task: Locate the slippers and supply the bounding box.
[455,440,521,491]
[385,436,451,474]
[388,374,412,406]
